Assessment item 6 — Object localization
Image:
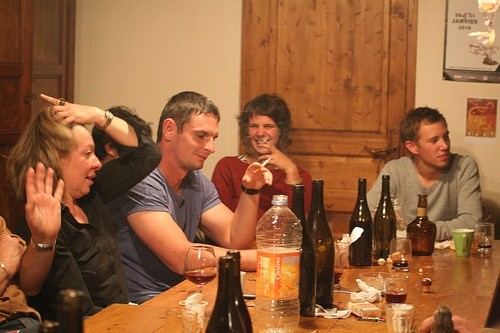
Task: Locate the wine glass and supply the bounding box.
[184,246,218,300]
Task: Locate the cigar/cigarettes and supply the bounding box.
[252,157,271,174]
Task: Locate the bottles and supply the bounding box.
[226,249,253,333]
[291,184,317,319]
[205,255,248,333]
[38,288,86,333]
[406,194,436,256]
[253,194,303,333]
[349,177,373,266]
[421,278,433,307]
[373,174,397,260]
[389,194,408,237]
[306,179,335,309]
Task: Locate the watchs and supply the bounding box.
[95,111,114,130]
[30,236,56,252]
[241,185,260,195]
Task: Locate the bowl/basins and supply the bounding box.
[359,272,391,296]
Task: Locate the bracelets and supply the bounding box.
[0,264,10,278]
[285,178,303,185]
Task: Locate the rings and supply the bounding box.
[58,98,66,105]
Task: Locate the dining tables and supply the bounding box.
[82,232,500,333]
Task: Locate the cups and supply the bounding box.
[476,222,494,258]
[390,238,412,272]
[333,238,343,290]
[180,300,208,333]
[384,272,409,303]
[165,309,197,333]
[451,229,475,257]
[385,303,415,333]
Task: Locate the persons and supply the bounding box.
[0,91,315,333]
[363,107,482,239]
